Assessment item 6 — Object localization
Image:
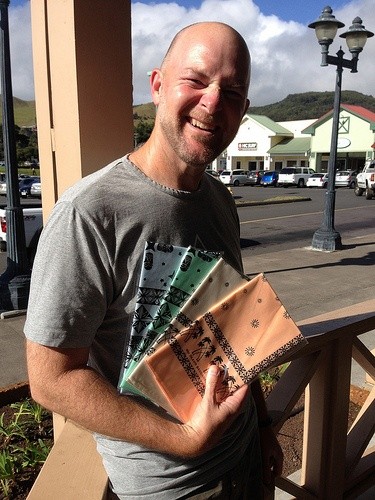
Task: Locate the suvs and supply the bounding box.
[246,170,269,185]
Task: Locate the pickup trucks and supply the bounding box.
[354,161,375,199]
[0,201,43,259]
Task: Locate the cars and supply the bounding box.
[306,172,329,189]
[0,173,41,199]
[0,160,39,169]
[259,171,279,187]
[204,165,224,179]
[334,170,359,189]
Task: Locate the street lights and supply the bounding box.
[307,6,375,252]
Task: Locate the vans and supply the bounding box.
[218,169,251,187]
[277,167,317,188]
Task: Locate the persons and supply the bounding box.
[23,21,285,500]
